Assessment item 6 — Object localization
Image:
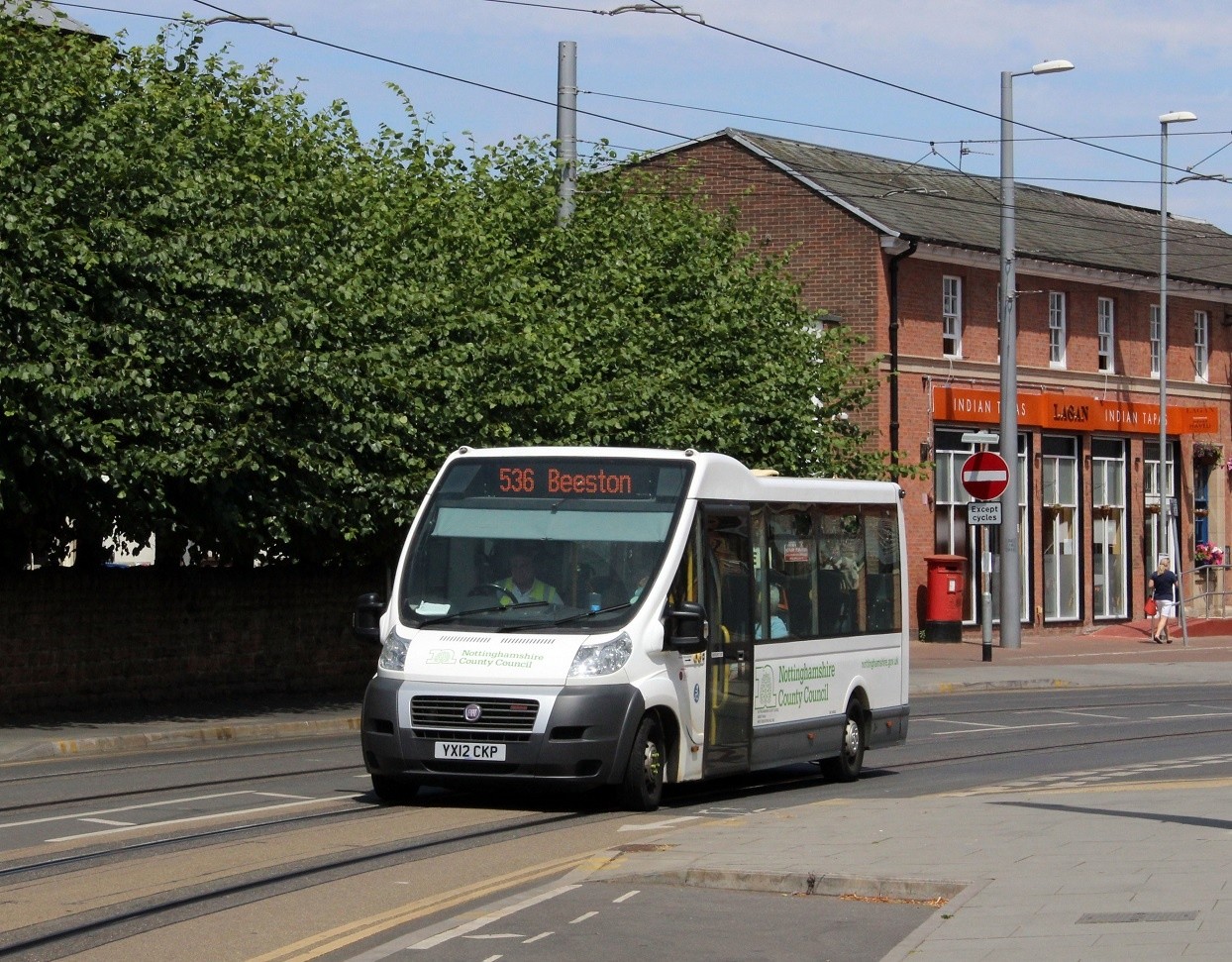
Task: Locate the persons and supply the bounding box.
[471,547,568,611]
[749,570,790,641]
[1149,557,1179,644]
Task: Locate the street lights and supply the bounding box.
[1158,111,1200,562]
[995,57,1077,649]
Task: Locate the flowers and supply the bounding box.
[1196,542,1223,565]
[1193,443,1225,470]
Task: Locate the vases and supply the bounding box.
[1194,451,1217,466]
[1195,561,1213,571]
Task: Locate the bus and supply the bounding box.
[360,444,911,812]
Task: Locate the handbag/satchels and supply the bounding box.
[1144,595,1157,616]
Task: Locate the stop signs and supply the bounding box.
[959,451,1011,502]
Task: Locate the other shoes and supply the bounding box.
[1166,640,1173,644]
[1154,636,1162,644]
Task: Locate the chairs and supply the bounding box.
[533,554,893,640]
